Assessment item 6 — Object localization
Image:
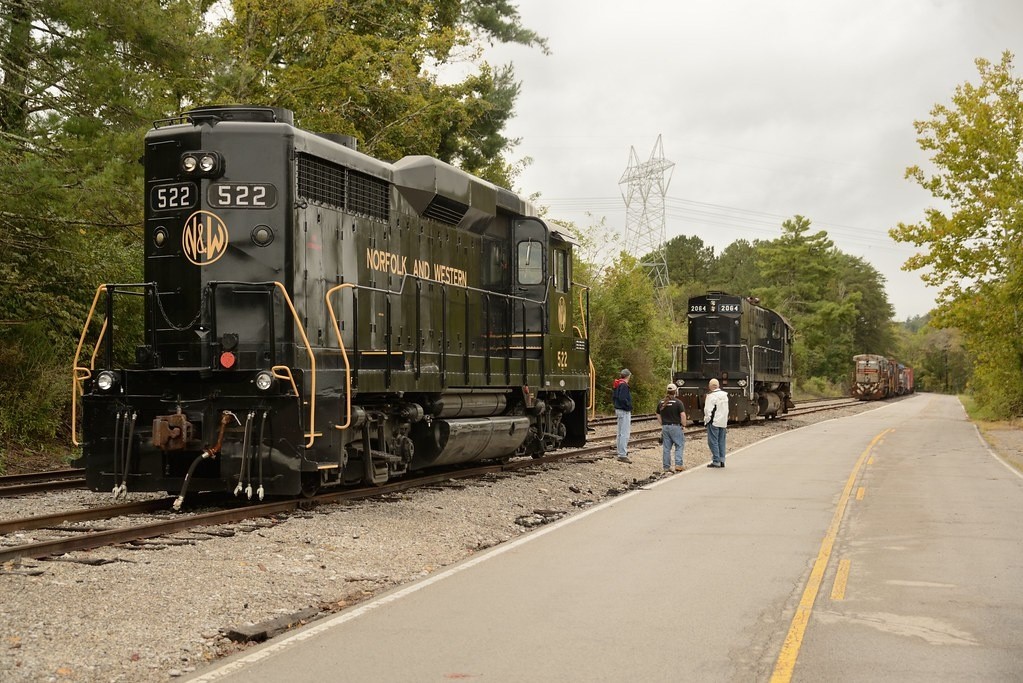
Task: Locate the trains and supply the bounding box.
[850,352,915,399]
[666,288,795,425]
[63,106,596,515]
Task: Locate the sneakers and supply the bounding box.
[618,456,632,463]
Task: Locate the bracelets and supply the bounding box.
[681,425,687,427]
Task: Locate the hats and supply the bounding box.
[709,379,719,387]
[620,369,631,376]
[667,384,676,391]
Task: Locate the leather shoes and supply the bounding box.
[707,462,724,468]
[675,466,685,471]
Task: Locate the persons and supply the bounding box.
[703,378,730,468]
[655,383,687,472]
[614,369,635,464]
